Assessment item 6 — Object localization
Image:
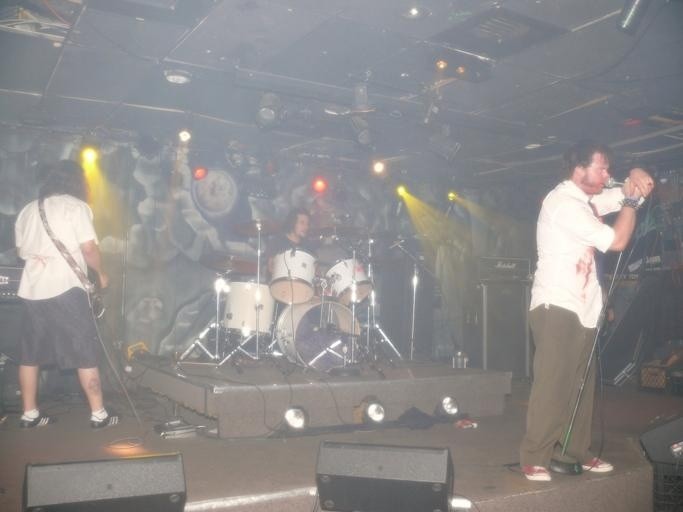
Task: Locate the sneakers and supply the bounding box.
[579,455,613,473]
[520,464,551,481]
[89,412,123,430]
[19,410,57,429]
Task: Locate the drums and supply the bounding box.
[200,249,258,279]
[320,256,373,311]
[275,295,361,375]
[269,247,319,306]
[219,278,278,339]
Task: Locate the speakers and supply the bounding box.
[640,416,683,464]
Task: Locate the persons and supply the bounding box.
[519,143,655,482]
[15,161,121,429]
[265,208,317,284]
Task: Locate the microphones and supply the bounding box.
[256,218,262,231]
[604,178,624,187]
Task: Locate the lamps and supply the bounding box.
[179,153,208,180]
[78,134,101,166]
[416,38,498,82]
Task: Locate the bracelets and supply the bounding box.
[620,198,639,211]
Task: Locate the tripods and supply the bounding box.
[177,231,433,370]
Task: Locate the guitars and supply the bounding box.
[87,267,106,323]
